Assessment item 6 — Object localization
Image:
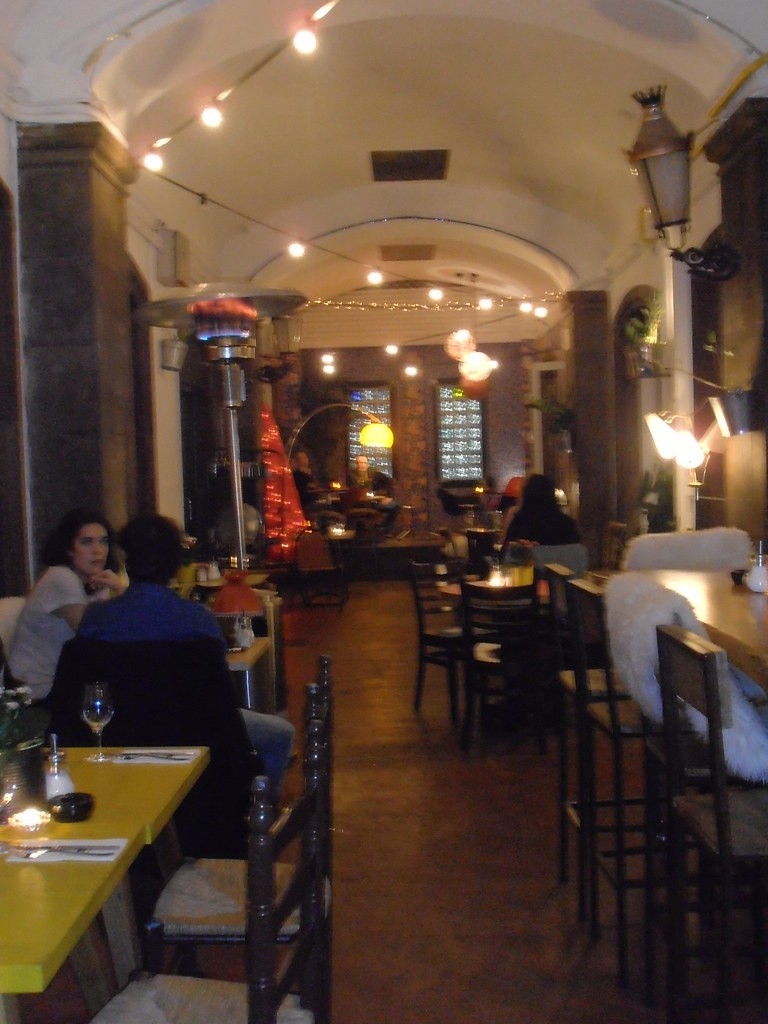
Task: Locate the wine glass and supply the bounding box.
[80,679,115,762]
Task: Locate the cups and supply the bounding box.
[42,752,76,801]
[207,561,219,579]
[237,616,253,648]
[196,566,207,581]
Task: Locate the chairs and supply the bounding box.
[76,477,768,1024]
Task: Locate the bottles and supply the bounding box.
[749,555,768,593]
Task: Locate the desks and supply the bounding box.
[585,554,767,691]
[440,560,567,702]
[219,634,274,711]
[174,573,268,588]
[1,747,209,1024]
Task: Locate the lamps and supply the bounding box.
[640,386,756,508]
[623,81,744,278]
[288,402,395,464]
[208,568,263,649]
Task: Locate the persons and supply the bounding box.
[502,473,584,546]
[8,510,129,707]
[293,450,322,505]
[76,515,294,821]
[347,455,380,487]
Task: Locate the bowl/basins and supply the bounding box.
[47,793,95,823]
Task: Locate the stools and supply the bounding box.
[614,573,753,1009]
[652,626,767,1024]
[544,561,632,923]
[567,578,702,990]
